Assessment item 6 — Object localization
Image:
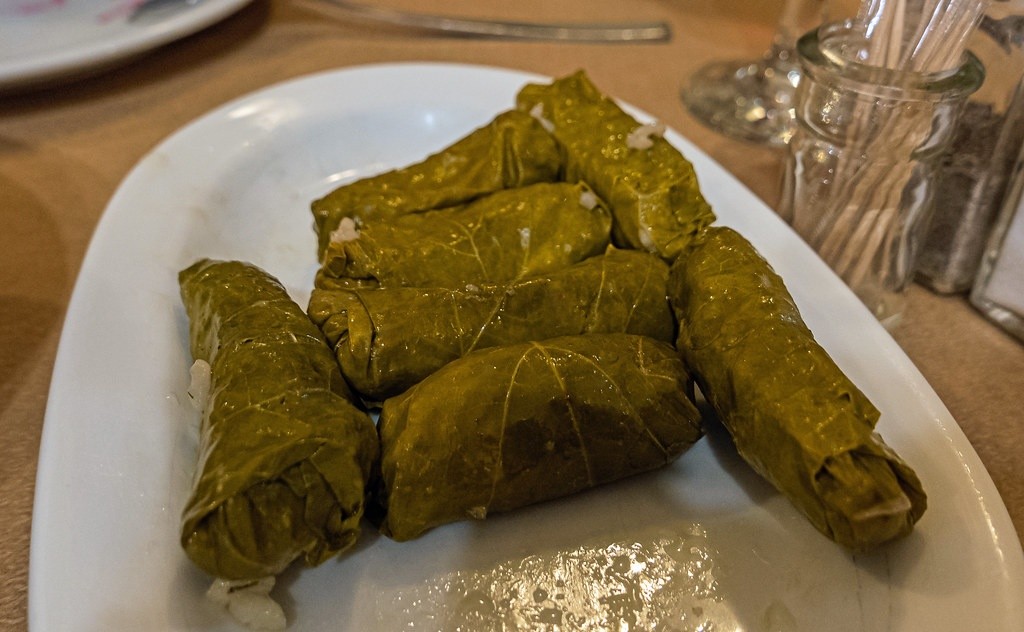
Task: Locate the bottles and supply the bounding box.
[916,66,1024,342]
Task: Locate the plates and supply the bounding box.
[27,58,1023,632]
[0,2,249,95]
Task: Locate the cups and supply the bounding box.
[775,18,988,329]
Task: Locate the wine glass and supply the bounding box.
[680,0,836,152]
